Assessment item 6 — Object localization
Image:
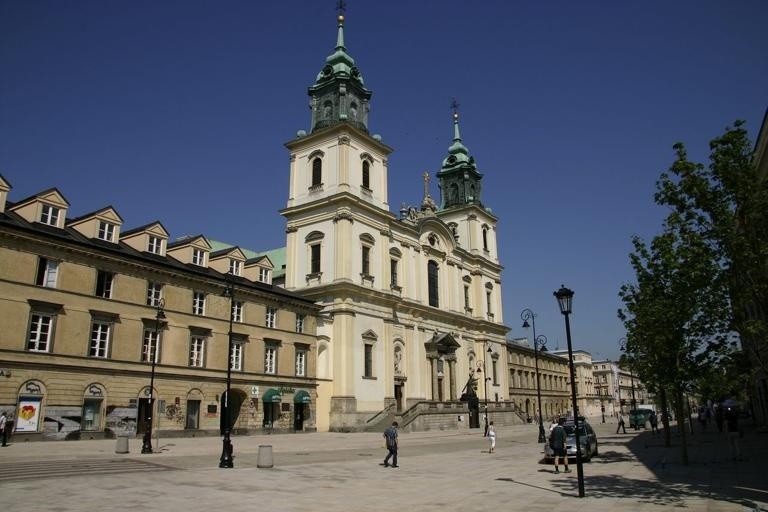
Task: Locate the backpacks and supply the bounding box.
[549,425,564,451]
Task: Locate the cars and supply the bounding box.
[629,409,652,427]
[544,417,597,464]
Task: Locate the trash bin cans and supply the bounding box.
[527,416,533,423]
[257,445,274,468]
[115,436,128,454]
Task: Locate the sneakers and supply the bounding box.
[383,460,399,468]
[554,469,573,474]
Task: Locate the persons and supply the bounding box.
[698,406,725,432]
[549,417,573,474]
[486,420,496,453]
[649,411,661,437]
[616,411,626,434]
[0,411,8,447]
[383,421,400,468]
[724,411,744,462]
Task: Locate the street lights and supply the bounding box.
[476,339,493,437]
[141,296,166,454]
[553,284,584,499]
[619,337,640,430]
[521,308,547,443]
[218,271,234,467]
